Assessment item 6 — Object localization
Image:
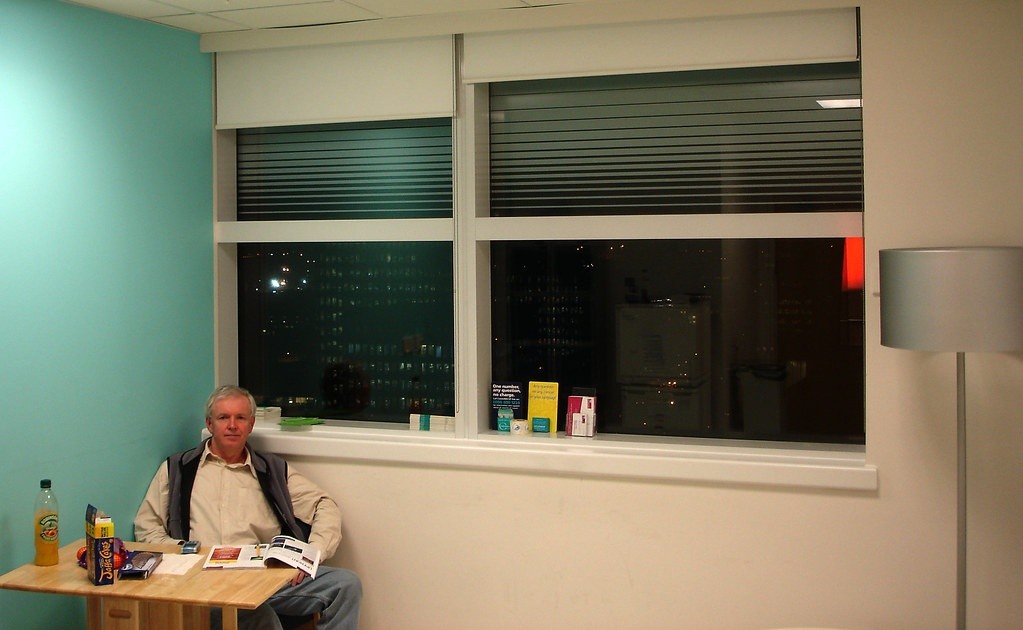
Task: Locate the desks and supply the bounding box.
[0,536,300,630]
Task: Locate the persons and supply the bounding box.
[134,385,362,630]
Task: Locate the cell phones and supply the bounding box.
[181,540,201,554]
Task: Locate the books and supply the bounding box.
[203,536,321,580]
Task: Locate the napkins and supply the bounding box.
[152,553,204,575]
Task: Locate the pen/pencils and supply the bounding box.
[256,543,260,559]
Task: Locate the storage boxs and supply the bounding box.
[566,396,597,437]
[117,551,163,579]
[498,419,513,431]
[533,417,550,433]
[85,503,115,586]
[510,419,529,434]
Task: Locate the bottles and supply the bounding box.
[33,479,59,566]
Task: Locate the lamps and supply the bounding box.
[880,248,1023,630]
